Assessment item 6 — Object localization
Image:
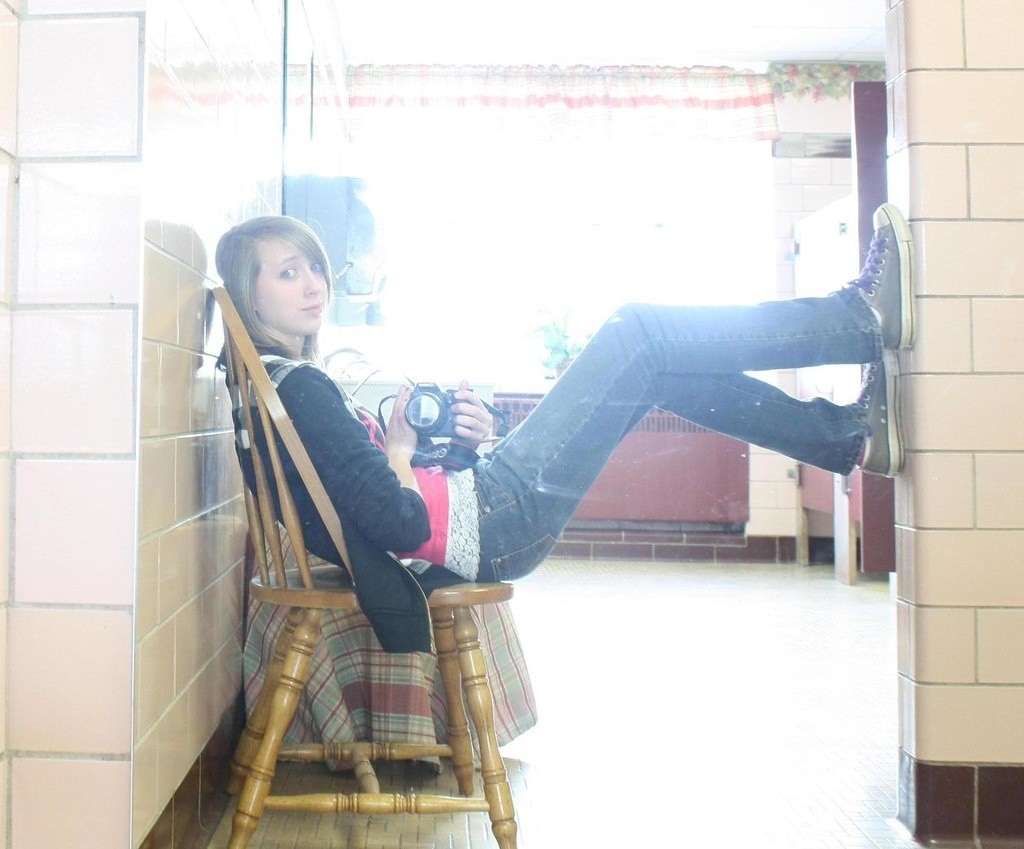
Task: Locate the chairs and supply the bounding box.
[214,285,517,849]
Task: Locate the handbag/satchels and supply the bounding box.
[411,442,483,471]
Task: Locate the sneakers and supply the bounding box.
[851,344,906,478]
[848,203,919,353]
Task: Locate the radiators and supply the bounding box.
[493,398,749,525]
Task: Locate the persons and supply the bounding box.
[215,205,917,653]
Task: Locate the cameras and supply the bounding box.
[405,382,473,441]
